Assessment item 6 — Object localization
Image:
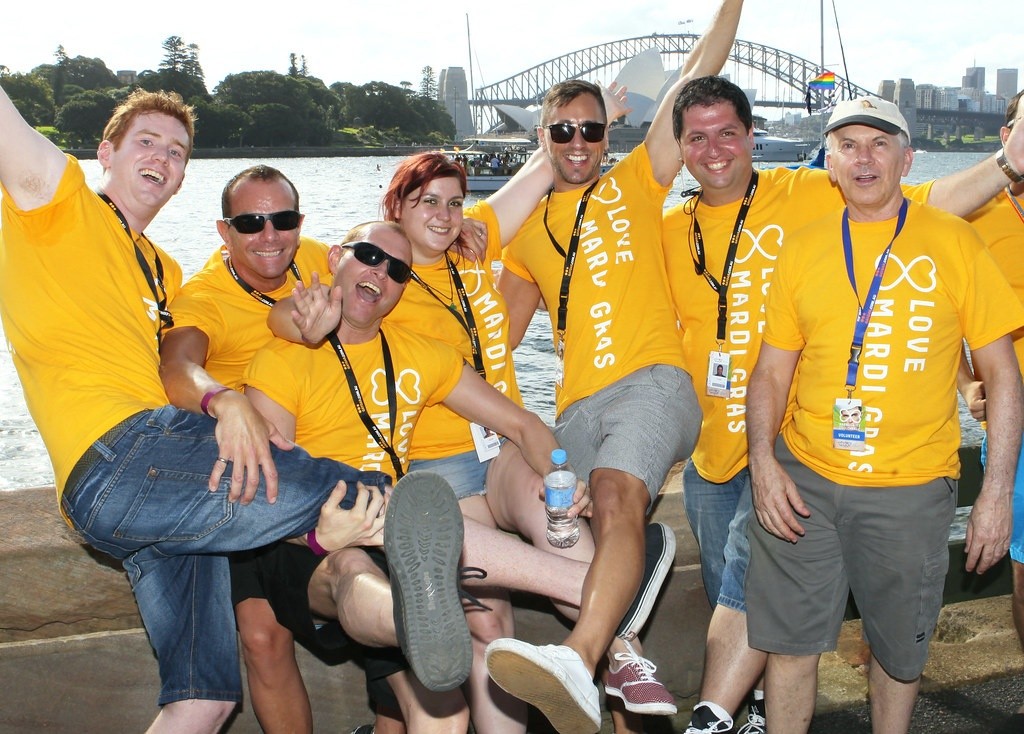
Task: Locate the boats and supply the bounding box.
[751,127,821,165]
[434,12,620,191]
[913,148,927,154]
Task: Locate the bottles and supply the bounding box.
[543,448,581,549]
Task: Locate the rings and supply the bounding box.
[478,231,484,237]
[215,457,229,465]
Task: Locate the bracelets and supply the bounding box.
[306,526,329,557]
[995,148,1024,184]
[201,386,233,420]
[299,334,325,348]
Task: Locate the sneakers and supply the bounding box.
[615,521,677,641]
[485,637,602,734]
[383,469,493,693]
[605,639,677,716]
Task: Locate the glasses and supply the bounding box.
[222,211,300,233]
[341,241,412,284]
[541,123,606,143]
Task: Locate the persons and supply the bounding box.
[498,0,745,734]
[174,146,684,734]
[0,92,677,734]
[748,94,1023,734]
[715,365,726,378]
[959,89,1023,649]
[483,427,494,439]
[646,77,1024,734]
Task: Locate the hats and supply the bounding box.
[823,96,910,146]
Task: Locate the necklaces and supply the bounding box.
[412,308,417,311]
[411,255,458,312]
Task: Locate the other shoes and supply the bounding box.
[736,690,766,734]
[351,724,374,734]
[682,705,734,734]
